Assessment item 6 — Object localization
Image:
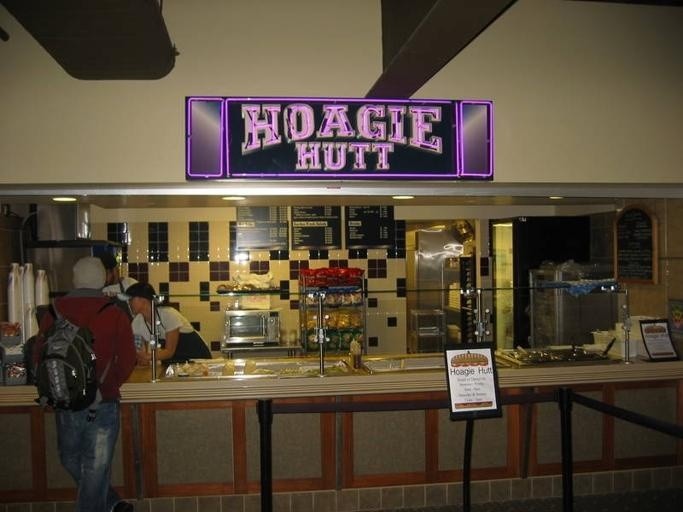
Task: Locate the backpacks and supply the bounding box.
[36,301,113,413]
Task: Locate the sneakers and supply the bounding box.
[112,501,133,512]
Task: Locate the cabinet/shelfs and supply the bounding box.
[441,258,461,346]
[410,308,447,353]
[298,272,368,355]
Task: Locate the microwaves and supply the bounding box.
[224,309,280,345]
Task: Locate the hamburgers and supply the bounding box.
[451,354,488,366]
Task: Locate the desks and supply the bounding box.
[221,346,302,358]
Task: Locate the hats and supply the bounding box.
[72,256,106,289]
[126,282,155,299]
[95,251,117,269]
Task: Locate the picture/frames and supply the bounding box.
[614,203,659,285]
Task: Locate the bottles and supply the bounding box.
[349,338,362,370]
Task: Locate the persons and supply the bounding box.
[94,250,139,322]
[125,282,212,368]
[31,255,136,512]
